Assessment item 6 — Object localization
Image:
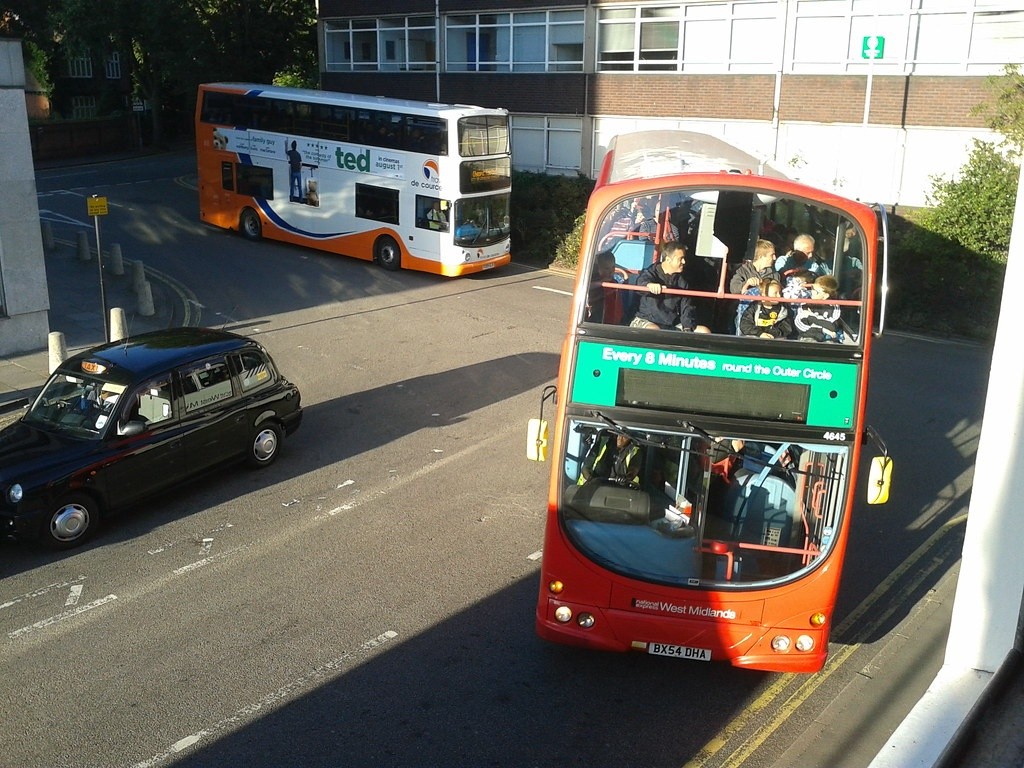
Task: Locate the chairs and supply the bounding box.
[77,357,261,426]
[205,99,448,157]
[575,428,803,540]
[595,200,834,338]
[424,208,433,229]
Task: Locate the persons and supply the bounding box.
[794,274,844,345]
[739,283,794,342]
[615,196,681,240]
[629,241,711,335]
[595,251,625,325]
[284,137,305,204]
[729,239,783,299]
[426,201,449,232]
[774,232,833,290]
[576,427,641,490]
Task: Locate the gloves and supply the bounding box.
[615,473,628,486]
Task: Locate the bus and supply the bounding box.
[525,127,890,675]
[193,79,513,278]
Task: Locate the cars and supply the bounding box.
[1,326,306,550]
